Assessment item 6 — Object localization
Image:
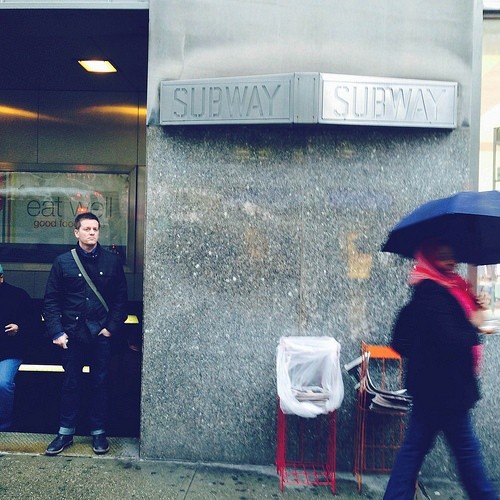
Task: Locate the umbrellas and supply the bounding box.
[379,190,500,266]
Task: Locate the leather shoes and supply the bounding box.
[92,433,110,453]
[47,434,74,454]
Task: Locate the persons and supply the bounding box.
[46,213,129,455]
[0,264,43,431]
[380,232,500,500]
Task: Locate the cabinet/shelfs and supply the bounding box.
[353,341,413,494]
[276,335,337,494]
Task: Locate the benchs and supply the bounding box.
[18,342,90,374]
